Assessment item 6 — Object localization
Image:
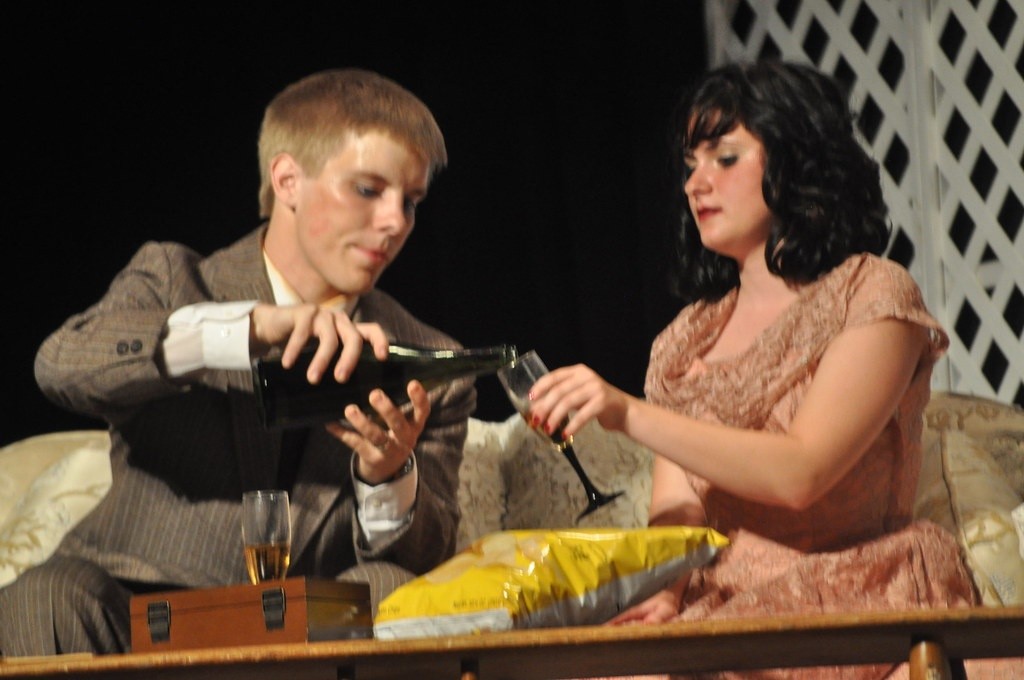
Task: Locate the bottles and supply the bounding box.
[286,340,517,426]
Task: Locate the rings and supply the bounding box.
[375,430,391,451]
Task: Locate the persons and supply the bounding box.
[0,71,479,680]
[527,60,979,680]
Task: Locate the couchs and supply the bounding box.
[0,390,1024,608]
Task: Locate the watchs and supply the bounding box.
[390,457,411,482]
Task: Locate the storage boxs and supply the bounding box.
[131,576,374,654]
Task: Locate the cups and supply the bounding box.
[241,492,292,585]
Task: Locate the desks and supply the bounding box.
[1,601,1024,680]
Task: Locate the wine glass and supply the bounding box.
[498,351,625,522]
